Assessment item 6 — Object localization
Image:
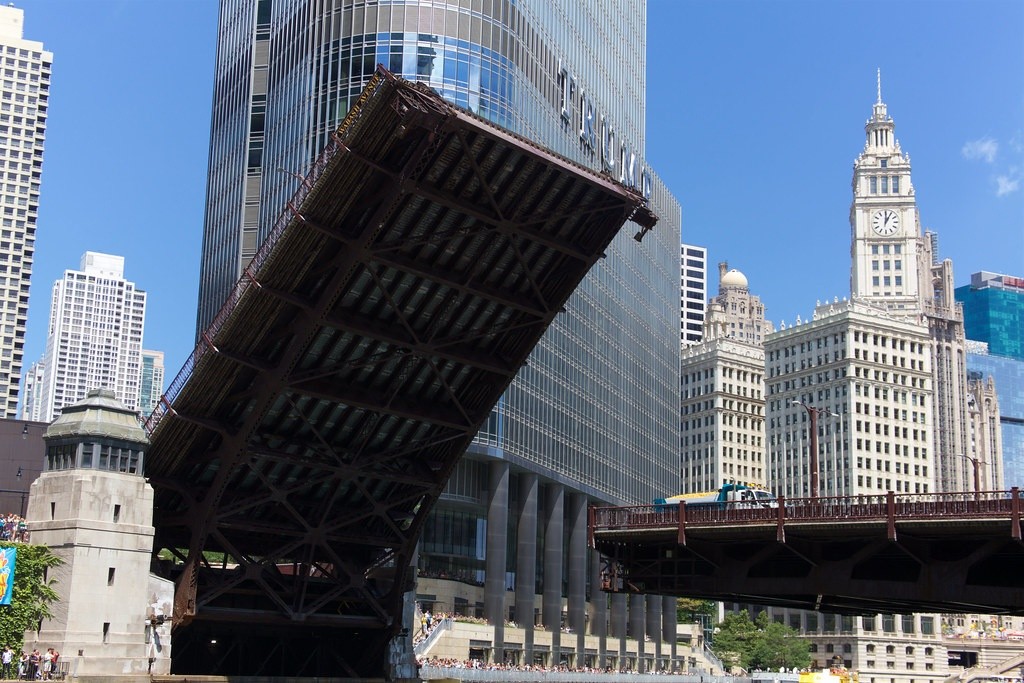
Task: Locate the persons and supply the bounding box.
[18,647,60,681]
[0,646,15,682]
[421,613,428,634]
[432,612,572,635]
[753,666,811,675]
[0,513,29,543]
[424,610,432,631]
[416,656,678,675]
[420,569,485,588]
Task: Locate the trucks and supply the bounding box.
[656,483,779,510]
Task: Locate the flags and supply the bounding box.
[0,546,16,606]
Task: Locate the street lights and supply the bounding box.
[791,400,840,504]
[956,453,993,500]
